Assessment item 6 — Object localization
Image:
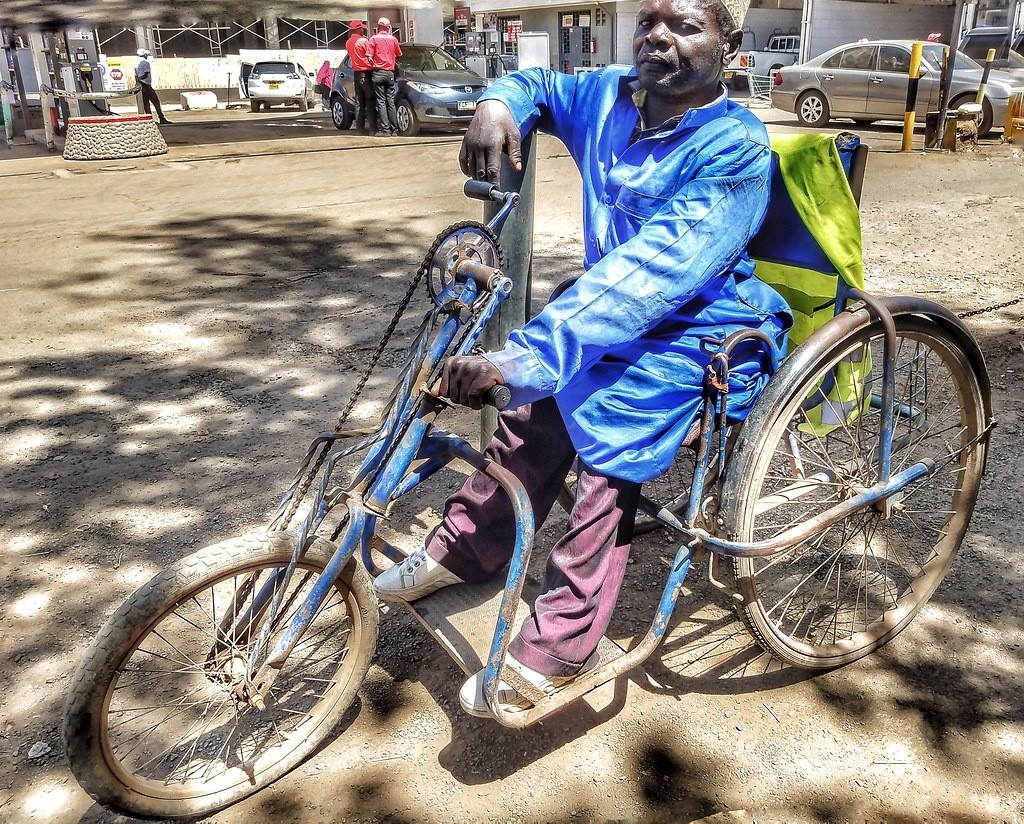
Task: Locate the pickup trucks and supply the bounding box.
[717,34,804,90]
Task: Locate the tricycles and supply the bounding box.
[53,110,1002,824]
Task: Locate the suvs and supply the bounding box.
[238,60,316,112]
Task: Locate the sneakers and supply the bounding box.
[372,545,465,602]
[459,651,599,719]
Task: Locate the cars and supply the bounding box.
[769,39,1023,137]
[329,42,491,135]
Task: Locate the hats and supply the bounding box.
[928,33,941,42]
[350,20,367,30]
[720,0,751,30]
[378,17,391,28]
[137,48,150,56]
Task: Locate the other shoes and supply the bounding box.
[375,131,391,137]
[160,118,172,124]
[155,122,158,124]
[369,131,377,136]
[392,133,397,137]
[357,129,368,135]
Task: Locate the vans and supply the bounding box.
[955,26,1023,74]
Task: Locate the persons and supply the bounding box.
[927,32,942,42]
[134,48,172,125]
[366,17,404,137]
[316,61,334,111]
[344,20,380,136]
[373,1,796,721]
